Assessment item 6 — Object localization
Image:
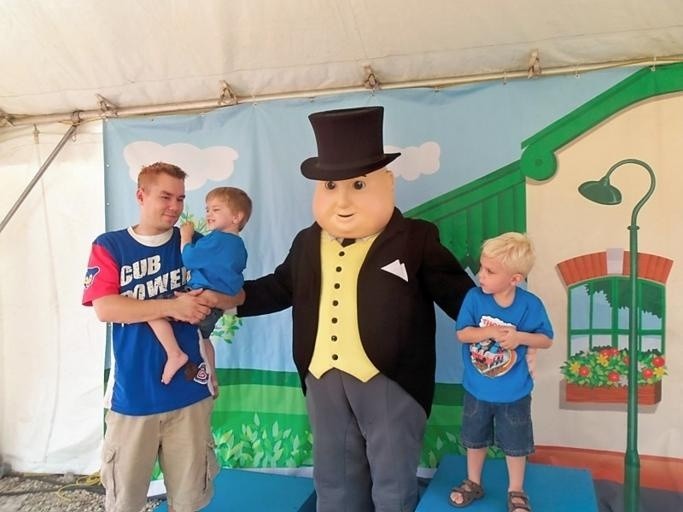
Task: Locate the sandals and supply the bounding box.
[508,490,530,511]
[448,479,484,507]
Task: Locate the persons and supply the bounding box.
[223,106,479,511]
[446,229,554,511]
[145,186,253,404]
[79,161,248,512]
